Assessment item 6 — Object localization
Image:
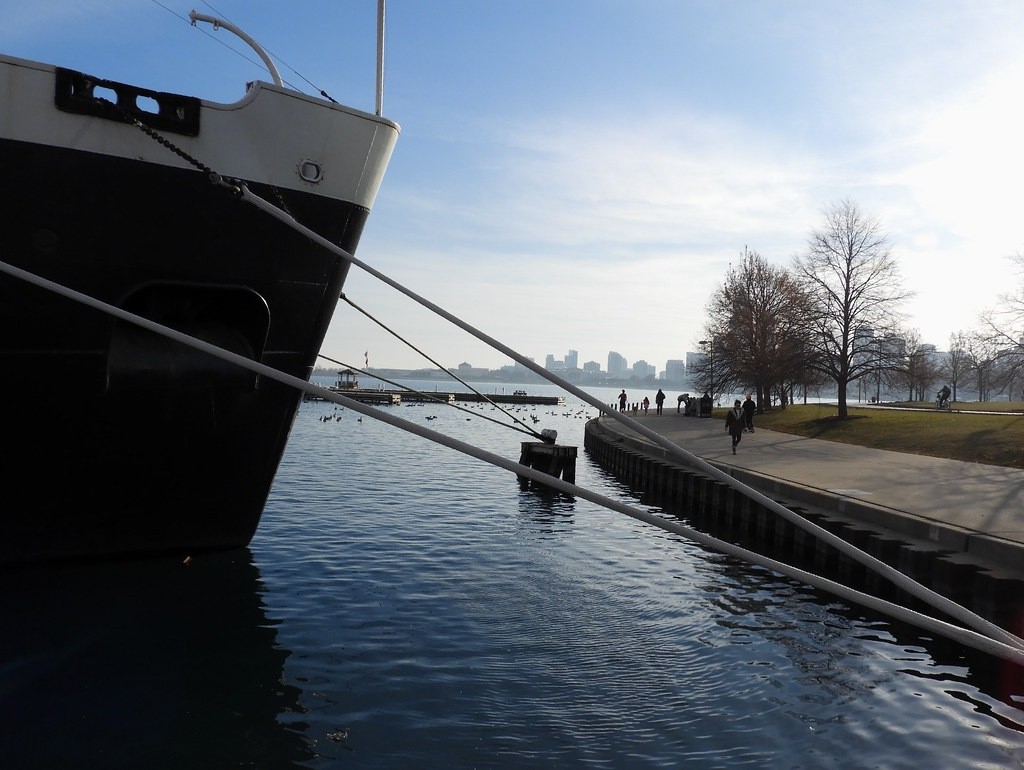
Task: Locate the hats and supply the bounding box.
[735,399,742,404]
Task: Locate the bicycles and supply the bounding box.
[934,392,951,411]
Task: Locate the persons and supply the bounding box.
[938,386,951,407]
[644,397,650,415]
[742,395,755,433]
[725,400,748,455]
[677,393,697,416]
[656,389,665,415]
[618,390,627,414]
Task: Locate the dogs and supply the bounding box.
[633,407,637,415]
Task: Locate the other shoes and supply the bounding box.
[733,448,736,455]
[751,427,754,433]
[735,441,737,446]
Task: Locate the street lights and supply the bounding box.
[699,340,713,417]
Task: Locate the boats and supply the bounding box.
[0,1,396,553]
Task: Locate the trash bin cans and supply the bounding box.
[696,398,712,417]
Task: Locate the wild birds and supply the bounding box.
[320,401,591,423]
